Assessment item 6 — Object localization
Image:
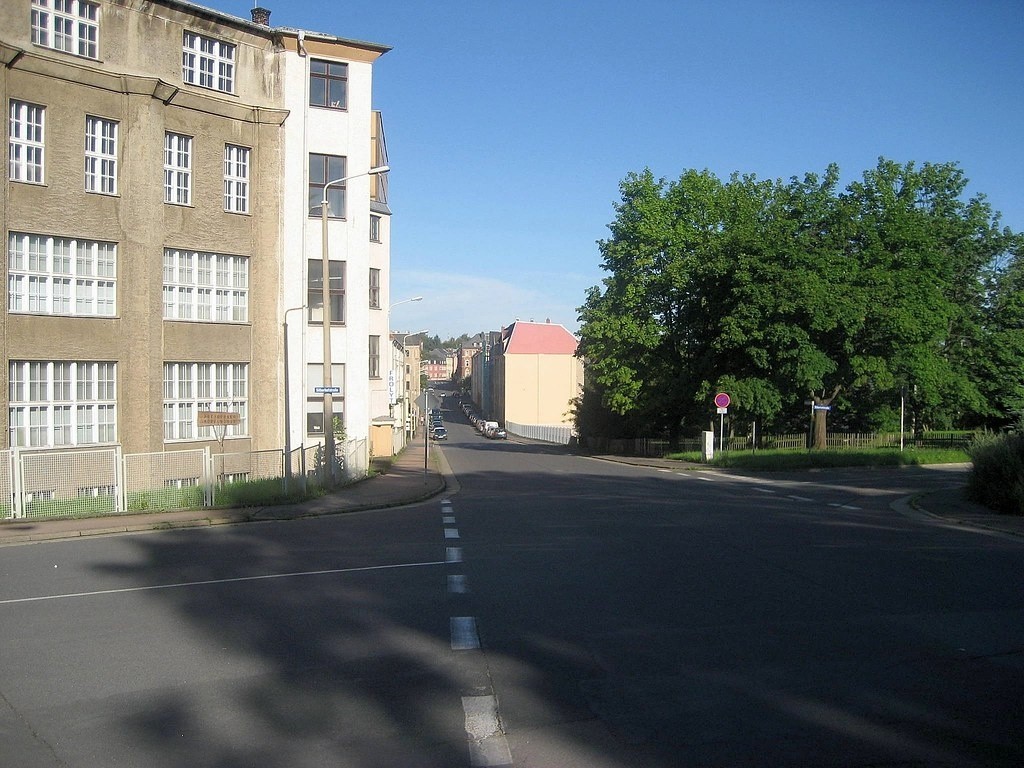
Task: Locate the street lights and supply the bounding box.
[321,165,391,488]
[402,330,430,449]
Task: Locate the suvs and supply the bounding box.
[431,427,449,441]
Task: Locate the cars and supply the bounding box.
[430,408,444,431]
[458,400,498,438]
[452,391,461,398]
[439,392,446,397]
[491,428,508,440]
